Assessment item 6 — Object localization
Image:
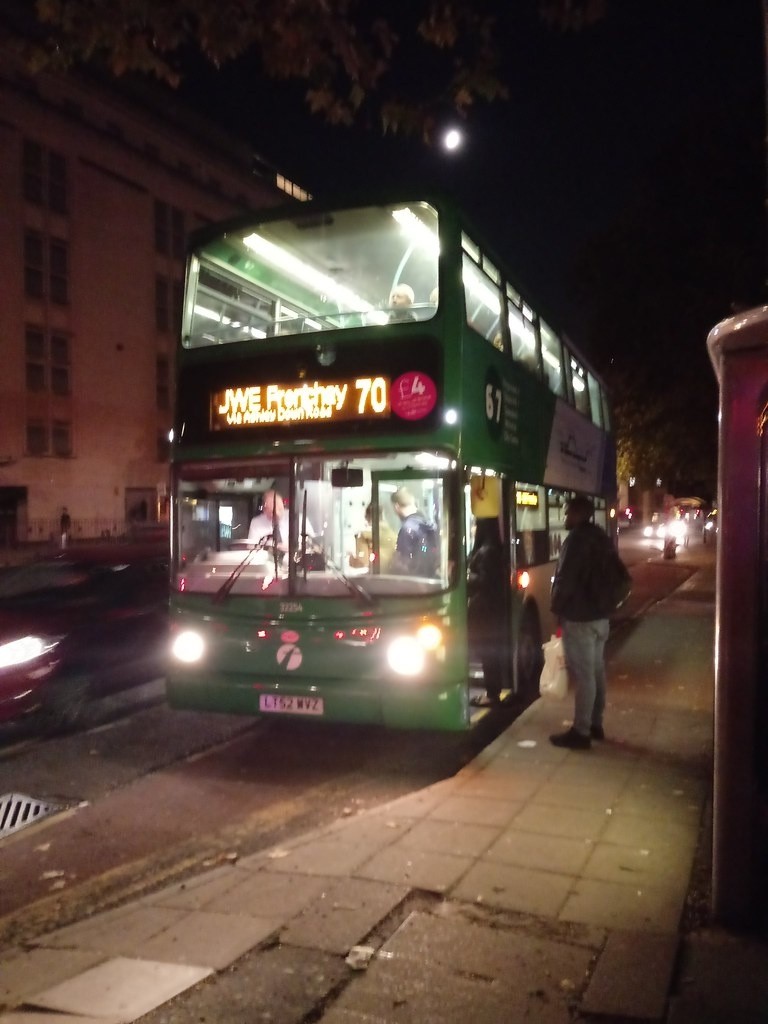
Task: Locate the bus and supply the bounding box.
[162,176,624,740]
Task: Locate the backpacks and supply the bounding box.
[413,519,441,576]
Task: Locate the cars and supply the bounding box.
[0,547,172,735]
[642,519,698,540]
[701,514,719,545]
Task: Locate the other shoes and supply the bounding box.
[590,726,605,740]
[549,731,591,749]
[469,695,501,707]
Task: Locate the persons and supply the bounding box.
[467,546,510,704]
[346,501,397,573]
[389,488,441,578]
[683,519,690,546]
[246,491,315,572]
[60,508,71,535]
[549,496,620,748]
